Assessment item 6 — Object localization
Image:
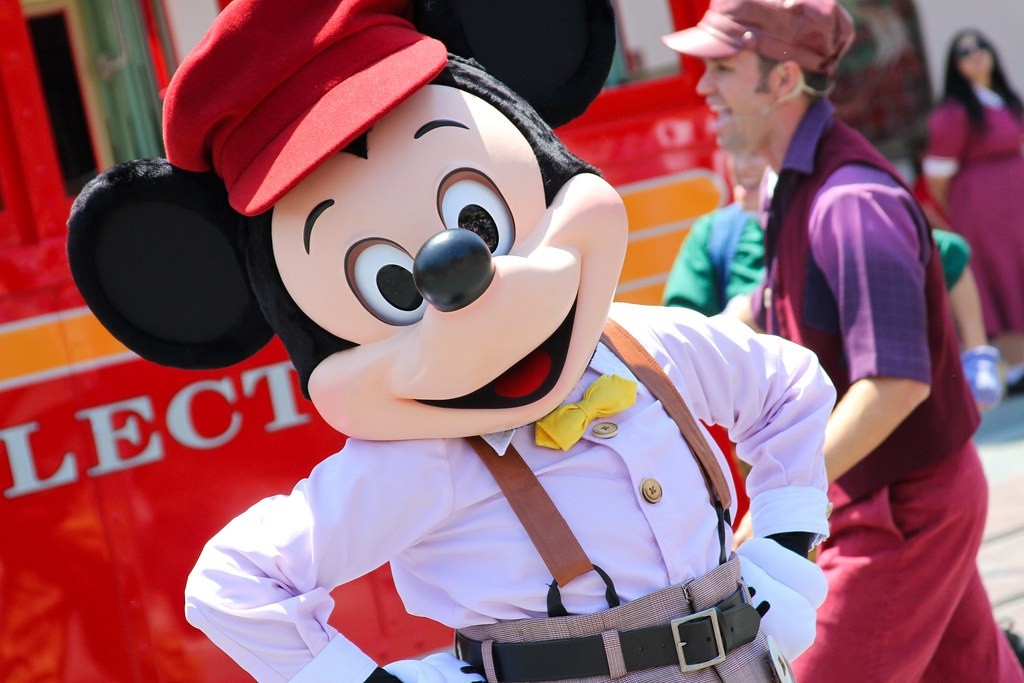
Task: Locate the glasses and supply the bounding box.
[956,41,984,57]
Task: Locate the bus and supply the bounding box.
[0,0,734,683]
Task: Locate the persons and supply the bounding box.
[662,146,1003,415]
[924,29,1024,364]
[663,0,1024,683]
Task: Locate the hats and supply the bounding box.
[662,0,855,75]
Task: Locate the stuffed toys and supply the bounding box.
[67,0,838,683]
